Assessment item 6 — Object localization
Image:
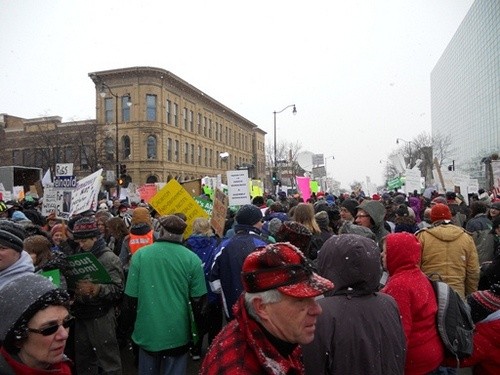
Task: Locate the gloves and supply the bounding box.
[75,280,98,299]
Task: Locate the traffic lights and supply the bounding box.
[120,165,126,179]
[272,172,276,180]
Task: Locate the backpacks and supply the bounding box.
[424,273,476,360]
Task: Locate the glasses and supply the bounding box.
[260,218,264,223]
[23,315,77,336]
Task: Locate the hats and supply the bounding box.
[152,215,188,243]
[341,199,359,217]
[0,220,26,252]
[51,224,68,236]
[326,195,335,201]
[465,284,500,323]
[267,217,282,235]
[132,206,151,226]
[316,192,324,198]
[431,196,449,206]
[46,214,57,221]
[0,272,70,354]
[490,202,500,210]
[240,242,335,299]
[235,204,263,226]
[193,218,210,233]
[23,235,51,254]
[396,204,409,216]
[12,210,25,221]
[314,211,329,227]
[471,201,487,213]
[430,204,453,223]
[446,191,456,198]
[72,217,99,240]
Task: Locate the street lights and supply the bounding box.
[100,82,133,198]
[380,160,389,180]
[396,138,411,158]
[273,104,297,194]
[325,155,334,167]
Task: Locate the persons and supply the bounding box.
[201,242,335,375]
[0,186,500,375]
